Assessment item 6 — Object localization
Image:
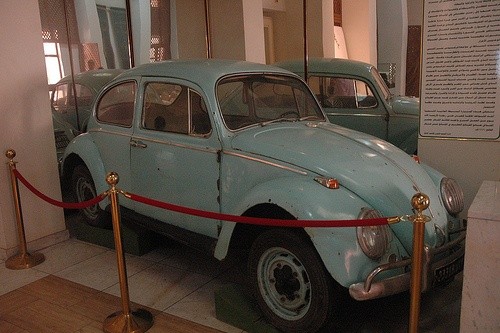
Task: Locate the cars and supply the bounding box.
[60,58,468,333]
[216,52,421,158]
[51,67,129,179]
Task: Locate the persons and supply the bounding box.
[88,59,95,71]
